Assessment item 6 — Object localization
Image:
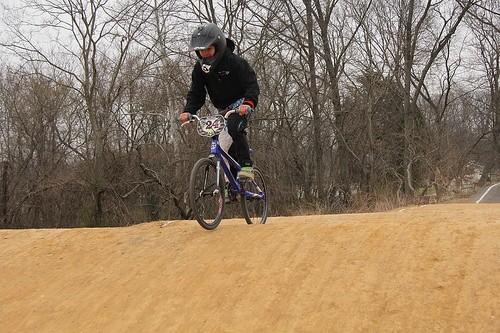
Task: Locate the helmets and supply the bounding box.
[188,21,226,74]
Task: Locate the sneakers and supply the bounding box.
[218,190,236,205]
[236,158,255,179]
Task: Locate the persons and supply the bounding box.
[178,22,261,203]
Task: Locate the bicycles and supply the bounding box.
[177,107,268,230]
